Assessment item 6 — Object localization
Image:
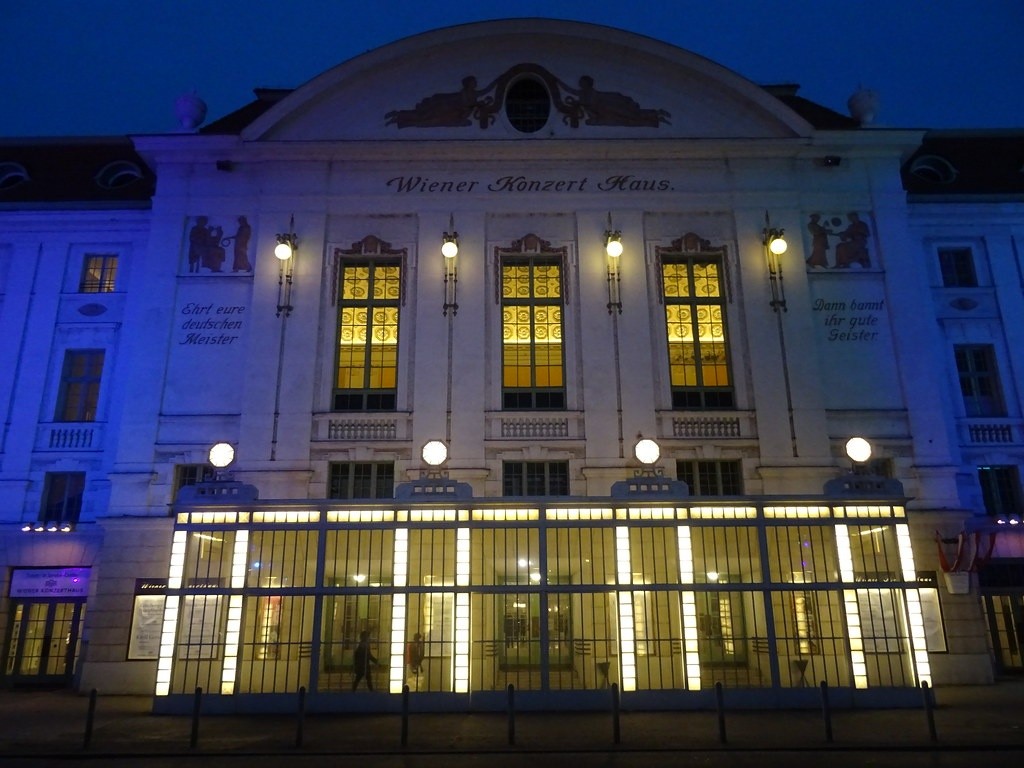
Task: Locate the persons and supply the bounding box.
[351,630,382,693]
[406,633,425,674]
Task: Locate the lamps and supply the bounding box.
[762,227,787,255]
[441,232,459,258]
[842,435,875,476]
[420,439,450,478]
[20,521,71,532]
[604,230,623,257]
[207,440,237,481]
[274,233,298,259]
[632,437,664,478]
